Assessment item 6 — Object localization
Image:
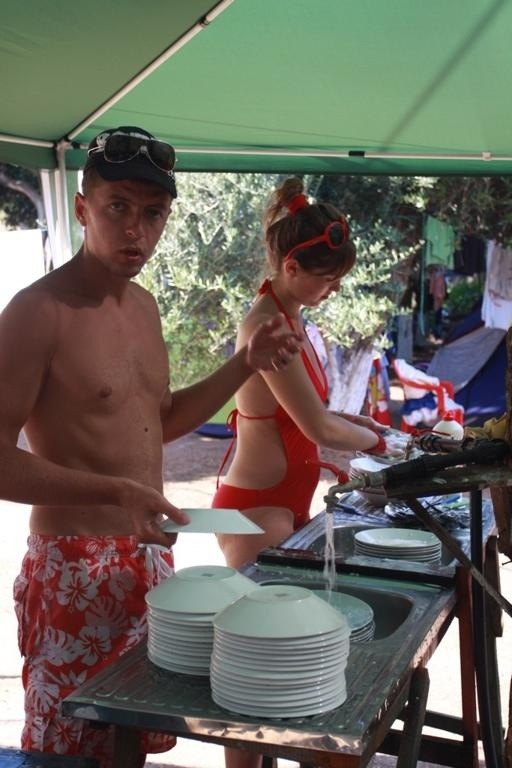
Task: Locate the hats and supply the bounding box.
[87,125,177,198]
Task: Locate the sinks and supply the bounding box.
[255,579,412,643]
[304,525,462,567]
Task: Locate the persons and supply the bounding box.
[0,126,306,768]
[213,176,417,768]
[424,264,451,325]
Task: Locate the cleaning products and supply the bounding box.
[433,412,465,510]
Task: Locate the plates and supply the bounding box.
[352,527,443,566]
[308,588,376,643]
[157,508,265,536]
[143,564,262,678]
[347,457,404,505]
[367,434,425,465]
[210,586,354,721]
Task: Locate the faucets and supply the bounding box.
[323,433,512,514]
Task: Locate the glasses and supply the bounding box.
[104,135,176,173]
[283,215,350,263]
